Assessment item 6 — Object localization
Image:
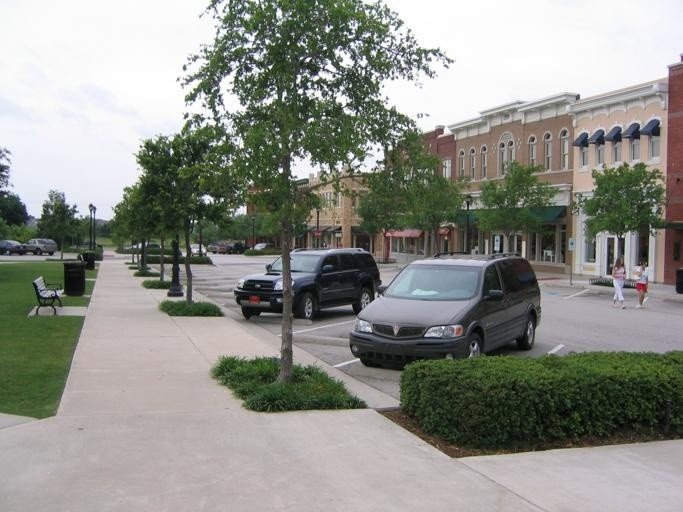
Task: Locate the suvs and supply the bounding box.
[22,239,57,255]
[233,247,382,320]
[349,252,542,370]
[132,243,160,249]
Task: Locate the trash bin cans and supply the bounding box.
[63,262,87,296]
[82,253,96,269]
[676,269,683,294]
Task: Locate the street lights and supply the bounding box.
[252,215,255,249]
[89,204,96,251]
[464,195,472,252]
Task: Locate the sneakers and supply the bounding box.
[612,303,644,309]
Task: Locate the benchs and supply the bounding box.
[31,275,64,316]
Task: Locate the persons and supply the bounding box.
[611,257,627,309]
[323,241,326,248]
[475,245,478,254]
[633,262,648,309]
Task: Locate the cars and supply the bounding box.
[250,242,273,250]
[0,240,27,255]
[190,244,207,256]
[209,242,250,254]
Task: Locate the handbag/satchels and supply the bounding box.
[632,273,641,279]
